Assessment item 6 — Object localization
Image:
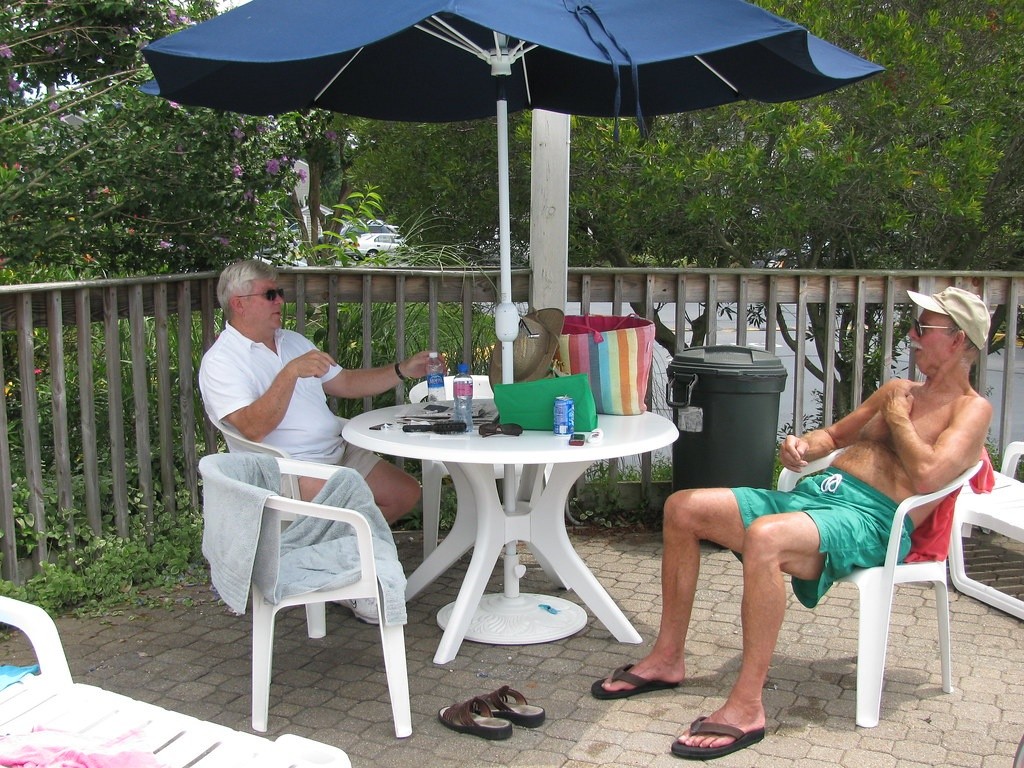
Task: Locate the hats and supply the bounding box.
[908,286,989,349]
[489,308,564,394]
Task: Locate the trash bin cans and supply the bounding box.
[664,343,788,552]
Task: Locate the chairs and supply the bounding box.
[0,596,351,768]
[198,454,412,739]
[208,416,349,639]
[777,446,983,728]
[948,441,1024,620]
[410,375,524,560]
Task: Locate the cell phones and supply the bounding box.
[424,404,451,413]
[569,434,585,446]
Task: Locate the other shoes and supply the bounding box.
[336,598,380,625]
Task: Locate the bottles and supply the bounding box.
[452,364,474,432]
[425,352,446,405]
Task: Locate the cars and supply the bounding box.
[337,216,409,261]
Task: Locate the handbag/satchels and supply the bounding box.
[552,313,655,415]
[493,372,598,431]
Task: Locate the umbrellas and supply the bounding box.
[134,0,890,648]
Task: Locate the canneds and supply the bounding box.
[553,395,575,437]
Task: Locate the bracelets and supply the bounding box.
[394,362,406,381]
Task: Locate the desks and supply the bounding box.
[342,400,679,664]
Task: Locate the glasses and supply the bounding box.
[914,317,945,335]
[479,422,523,437]
[238,288,286,301]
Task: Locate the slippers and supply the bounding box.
[439,697,512,739]
[670,714,766,759]
[591,663,679,699]
[478,685,545,728]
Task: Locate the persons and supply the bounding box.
[591,286,990,760]
[198,259,420,624]
